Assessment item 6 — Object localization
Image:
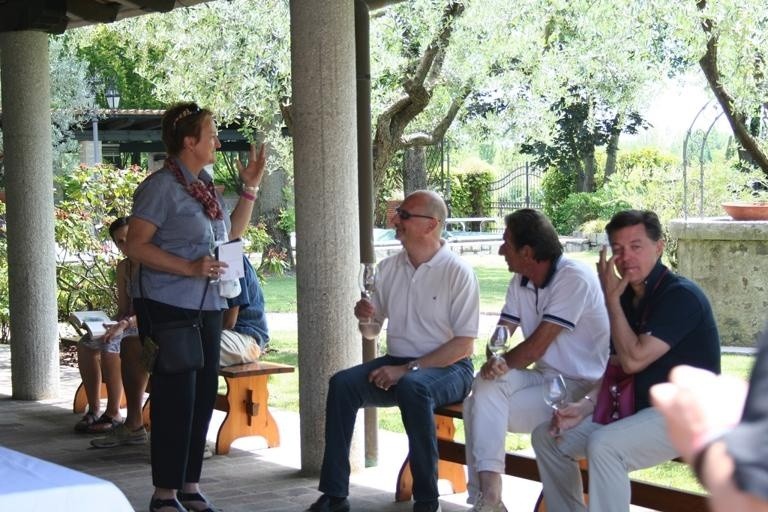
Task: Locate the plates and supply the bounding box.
[721,202,768,220]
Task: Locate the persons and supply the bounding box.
[649,340,764,511]
[531,209,721,511]
[90,246,270,459]
[125,102,267,511]
[461,208,610,511]
[305,191,478,511]
[74,215,136,435]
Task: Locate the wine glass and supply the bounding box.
[543,374,568,436]
[358,263,379,325]
[487,324,511,383]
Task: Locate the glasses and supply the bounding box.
[395,206,441,224]
[608,378,622,422]
[172,102,200,132]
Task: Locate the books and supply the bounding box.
[70,310,120,341]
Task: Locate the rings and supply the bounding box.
[208,273,212,278]
[210,267,214,271]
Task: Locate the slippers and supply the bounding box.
[74,412,100,432]
[88,413,125,434]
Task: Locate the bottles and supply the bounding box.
[208,240,224,286]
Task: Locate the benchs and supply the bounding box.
[60,338,294,454]
[395,404,710,512]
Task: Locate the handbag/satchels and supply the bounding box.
[593,360,637,424]
[139,318,206,376]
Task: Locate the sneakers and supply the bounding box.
[467,491,509,512]
[90,422,148,449]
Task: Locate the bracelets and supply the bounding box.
[123,315,131,328]
[240,192,258,201]
[585,394,597,412]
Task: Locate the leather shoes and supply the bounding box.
[412,499,438,512]
[303,496,350,512]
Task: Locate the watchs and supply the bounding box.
[240,184,260,194]
[407,358,422,373]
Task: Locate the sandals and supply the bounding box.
[150,494,190,512]
[177,490,223,512]
